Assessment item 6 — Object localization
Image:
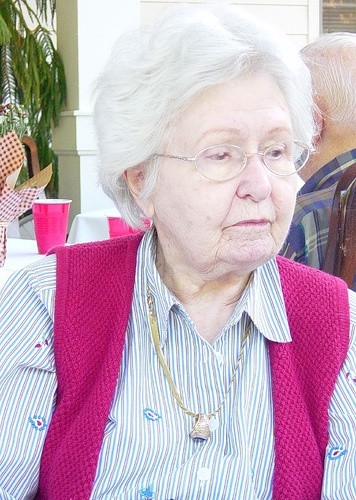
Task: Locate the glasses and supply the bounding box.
[152,138,310,182]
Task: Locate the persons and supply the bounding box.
[0,5,356,500]
[276,31,356,290]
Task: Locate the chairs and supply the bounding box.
[320,164,356,294]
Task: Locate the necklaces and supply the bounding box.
[146,291,252,441]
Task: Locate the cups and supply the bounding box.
[33,199,72,254]
[108,213,150,239]
